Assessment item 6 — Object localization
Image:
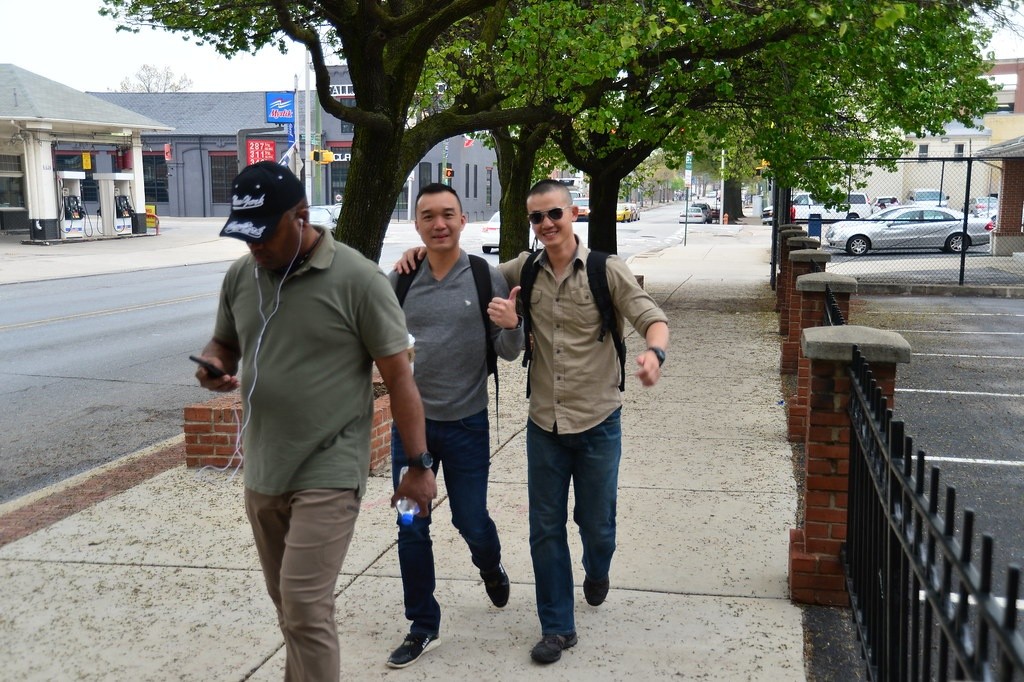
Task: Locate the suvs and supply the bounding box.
[692,203,714,224]
[573,198,589,221]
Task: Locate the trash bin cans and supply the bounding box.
[132,212,147,234]
[808,214,821,236]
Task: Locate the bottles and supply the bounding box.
[398,467,420,524]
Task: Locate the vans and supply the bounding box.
[760,192,871,226]
[906,188,949,207]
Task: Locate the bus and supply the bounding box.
[570,191,585,199]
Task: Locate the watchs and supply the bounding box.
[504,314,522,329]
[648,347,665,366]
[409,451,434,469]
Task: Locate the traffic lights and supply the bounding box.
[445,169,455,178]
[312,150,324,163]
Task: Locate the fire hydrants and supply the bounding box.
[723,213,729,226]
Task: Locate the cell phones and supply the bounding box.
[188,354,226,379]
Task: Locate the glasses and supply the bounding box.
[526,205,574,224]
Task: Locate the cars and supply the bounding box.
[984,210,999,223]
[961,197,999,215]
[616,204,632,222]
[482,211,539,254]
[824,207,993,257]
[711,208,720,219]
[679,207,708,225]
[869,197,901,215]
[628,203,642,221]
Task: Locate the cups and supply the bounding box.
[407,334,415,374]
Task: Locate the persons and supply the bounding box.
[384,184,527,668]
[968,198,977,213]
[195,159,436,682]
[879,203,886,209]
[891,197,899,205]
[946,196,950,205]
[875,201,881,206]
[393,180,669,664]
[907,196,914,205]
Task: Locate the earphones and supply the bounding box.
[298,218,303,224]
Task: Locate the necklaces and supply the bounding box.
[298,230,323,266]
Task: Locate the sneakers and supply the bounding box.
[479,563,511,607]
[385,632,442,668]
[583,575,609,606]
[530,631,578,663]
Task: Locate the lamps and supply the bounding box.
[941,138,948,142]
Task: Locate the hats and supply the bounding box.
[219,160,306,243]
